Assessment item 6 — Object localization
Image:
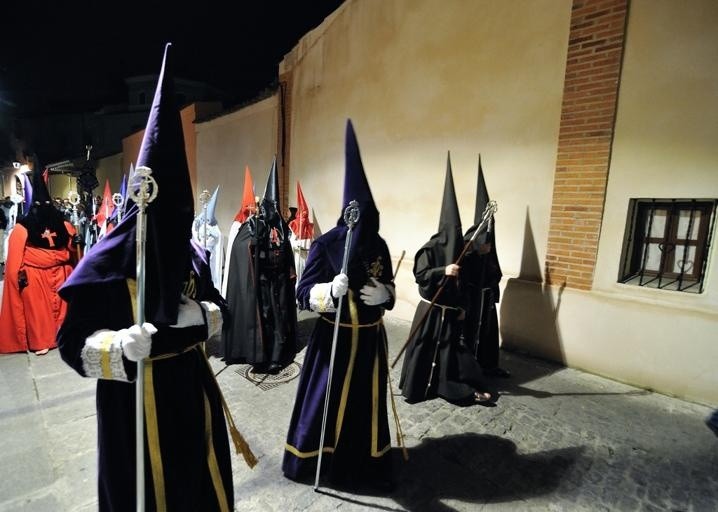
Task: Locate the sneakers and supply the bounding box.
[268,364,282,372]
[474,392,491,402]
[484,367,509,377]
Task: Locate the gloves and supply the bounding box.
[72,234,86,250]
[121,322,158,362]
[169,293,205,328]
[18,270,28,287]
[332,273,349,299]
[251,235,262,245]
[360,277,390,306]
